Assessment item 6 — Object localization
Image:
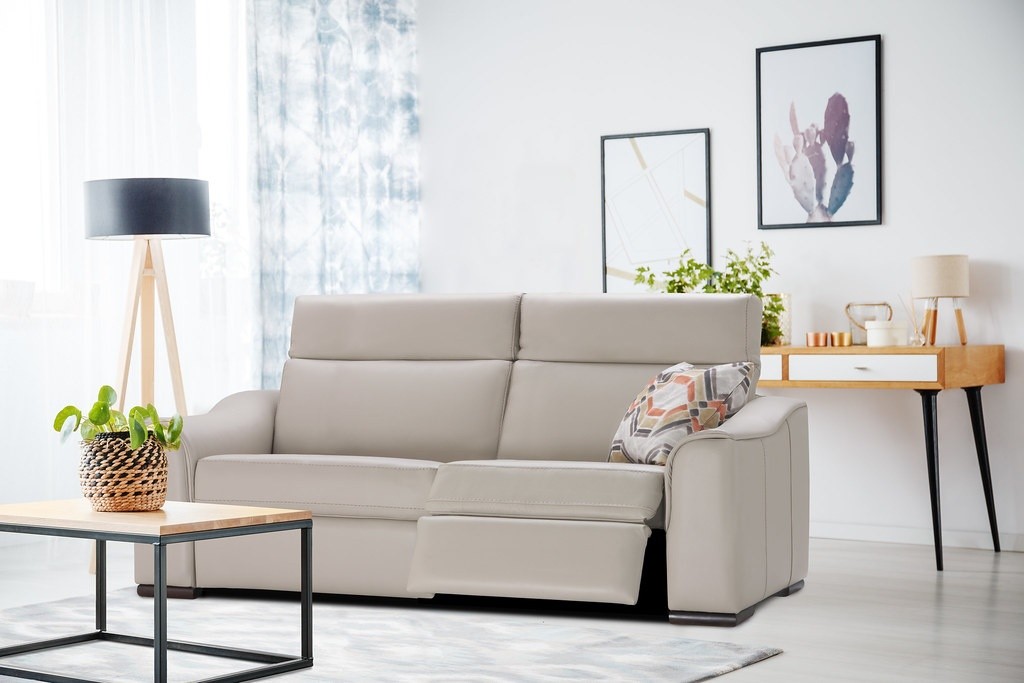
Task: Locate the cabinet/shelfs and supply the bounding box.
[755,342,1008,574]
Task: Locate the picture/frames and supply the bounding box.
[598,126,713,293]
[753,30,884,230]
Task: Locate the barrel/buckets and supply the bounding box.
[866,319,908,346]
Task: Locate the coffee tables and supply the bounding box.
[0,497,316,683]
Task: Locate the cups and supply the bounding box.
[806,331,828,347]
[831,332,852,346]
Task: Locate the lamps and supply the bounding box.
[82,179,211,414]
[908,255,972,345]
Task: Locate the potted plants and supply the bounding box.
[51,385,185,512]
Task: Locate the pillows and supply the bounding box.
[608,362,757,469]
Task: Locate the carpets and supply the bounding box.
[1,585,782,681]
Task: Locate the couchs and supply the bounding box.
[131,291,812,626]
